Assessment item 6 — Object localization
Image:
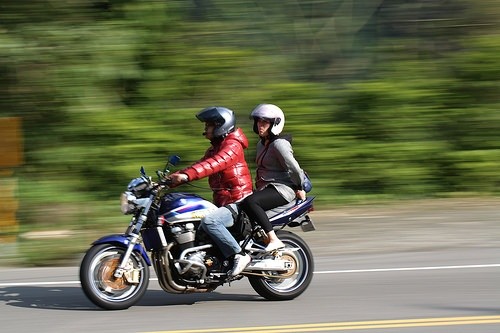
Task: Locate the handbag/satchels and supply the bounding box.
[303,171,312,194]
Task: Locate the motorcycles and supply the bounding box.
[78,154,318,312]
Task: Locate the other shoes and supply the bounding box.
[232,252,251,275]
[264,240,285,252]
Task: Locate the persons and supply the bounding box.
[162,106,253,276]
[244,103,306,252]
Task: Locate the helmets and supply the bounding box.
[195,106,236,140]
[249,104,285,135]
